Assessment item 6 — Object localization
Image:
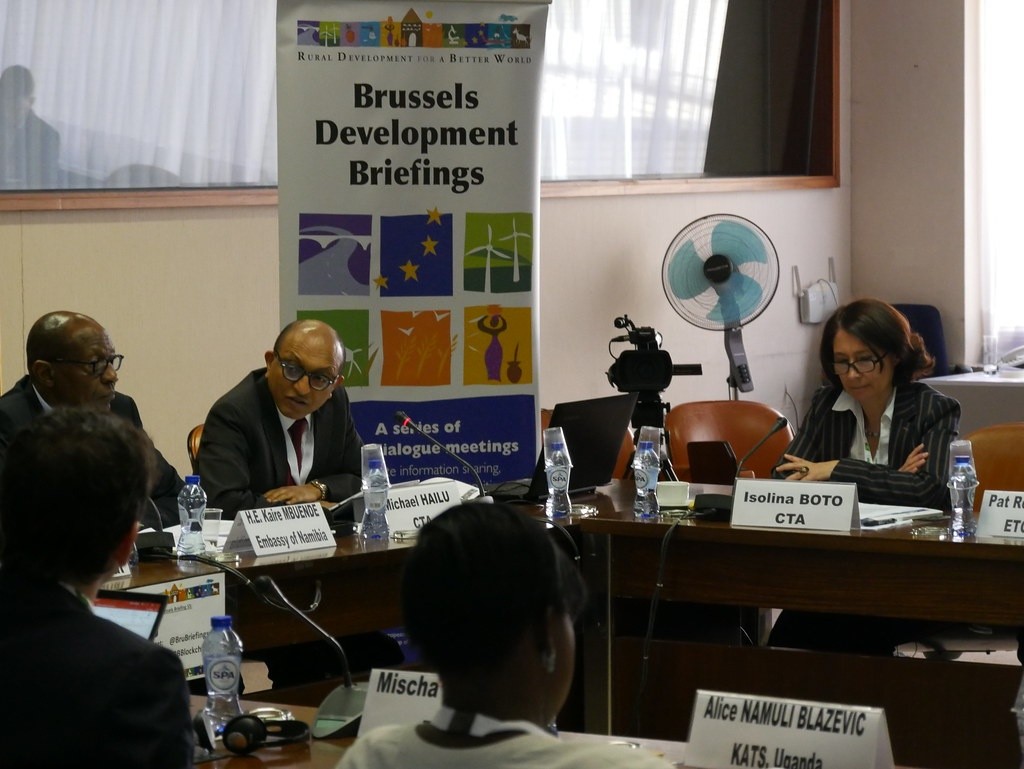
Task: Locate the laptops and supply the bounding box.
[483,391,642,504]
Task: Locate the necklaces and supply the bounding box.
[865,427,880,438]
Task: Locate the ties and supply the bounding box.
[287,418,308,476]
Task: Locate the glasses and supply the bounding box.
[49,354,124,376]
[277,351,340,391]
[832,350,889,376]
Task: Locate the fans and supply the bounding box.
[661,212,780,404]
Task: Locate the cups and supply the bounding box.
[946,439,980,490]
[360,443,393,492]
[202,508,223,550]
[543,426,574,472]
[983,336,998,374]
[630,425,661,472]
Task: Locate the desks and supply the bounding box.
[176,696,359,769]
[99,493,611,686]
[918,367,1024,440]
[578,490,1024,768]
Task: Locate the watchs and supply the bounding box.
[308,479,327,501]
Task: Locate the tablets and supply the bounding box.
[329,478,422,512]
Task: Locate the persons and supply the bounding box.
[769,298,961,654]
[193,320,405,688]
[0,311,187,530]
[335,502,675,769]
[0,405,195,769]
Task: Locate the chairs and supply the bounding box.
[184,300,1024,513]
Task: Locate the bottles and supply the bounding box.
[546,441,573,518]
[359,460,390,539]
[177,475,206,566]
[949,455,977,536]
[633,440,660,518]
[202,616,241,732]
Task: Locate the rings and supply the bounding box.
[799,465,809,476]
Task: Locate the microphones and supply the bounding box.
[394,410,486,499]
[693,418,788,519]
[129,551,371,740]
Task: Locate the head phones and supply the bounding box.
[193,709,311,753]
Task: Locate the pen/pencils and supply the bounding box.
[865,519,896,526]
